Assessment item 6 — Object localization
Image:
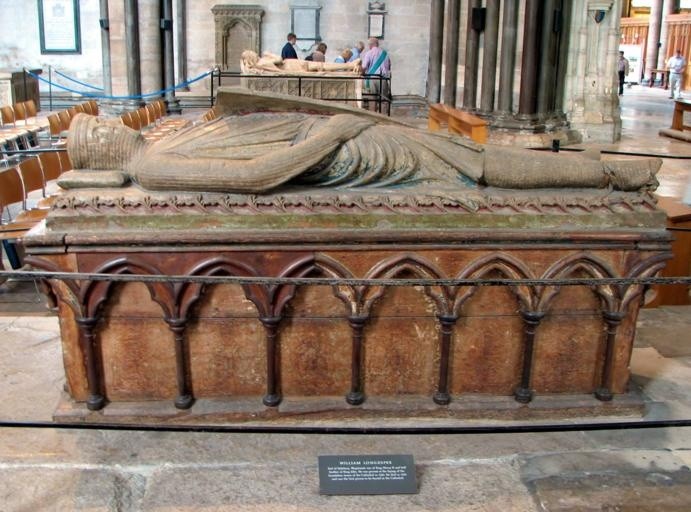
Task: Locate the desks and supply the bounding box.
[0,67,43,114]
[649,68,670,91]
[671,100,691,130]
[428,102,487,143]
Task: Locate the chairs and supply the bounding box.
[0,98,224,317]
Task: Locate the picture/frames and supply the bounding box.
[367,12,384,39]
[37,0,83,56]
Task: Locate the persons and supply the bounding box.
[348,42,365,110]
[335,49,353,72]
[281,33,298,61]
[68,110,665,195]
[362,37,392,117]
[312,43,327,63]
[617,51,629,96]
[666,50,687,100]
